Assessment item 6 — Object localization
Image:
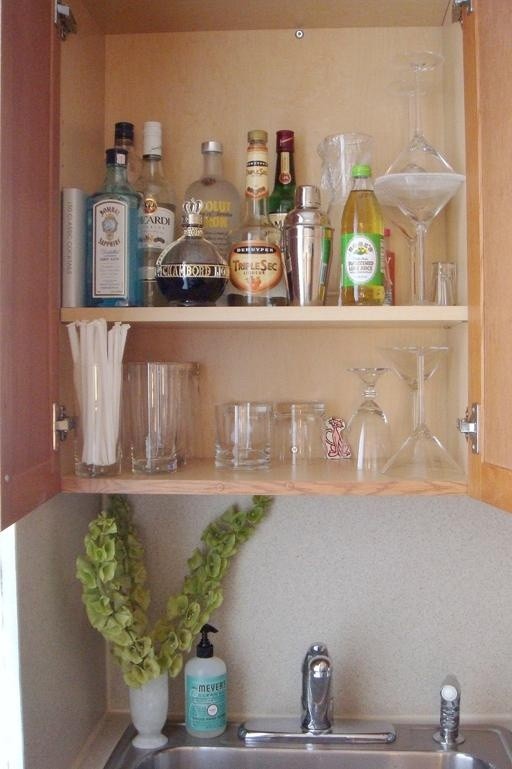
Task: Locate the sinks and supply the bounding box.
[101,719,512,769]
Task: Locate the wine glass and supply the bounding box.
[381,49,459,178]
[337,366,398,470]
[381,344,469,483]
[371,174,465,306]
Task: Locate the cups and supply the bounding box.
[212,400,273,475]
[67,359,128,479]
[272,401,332,465]
[124,359,204,478]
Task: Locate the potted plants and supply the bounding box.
[74,494,276,750]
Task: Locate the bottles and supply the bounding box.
[428,260,459,305]
[82,147,143,309]
[134,119,177,308]
[153,198,233,307]
[182,141,245,307]
[225,129,289,307]
[315,131,376,306]
[113,120,143,195]
[280,184,336,307]
[267,130,300,307]
[381,227,397,306]
[341,166,385,304]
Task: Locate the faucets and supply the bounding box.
[302,644,334,735]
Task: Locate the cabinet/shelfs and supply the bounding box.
[1,1,511,531]
